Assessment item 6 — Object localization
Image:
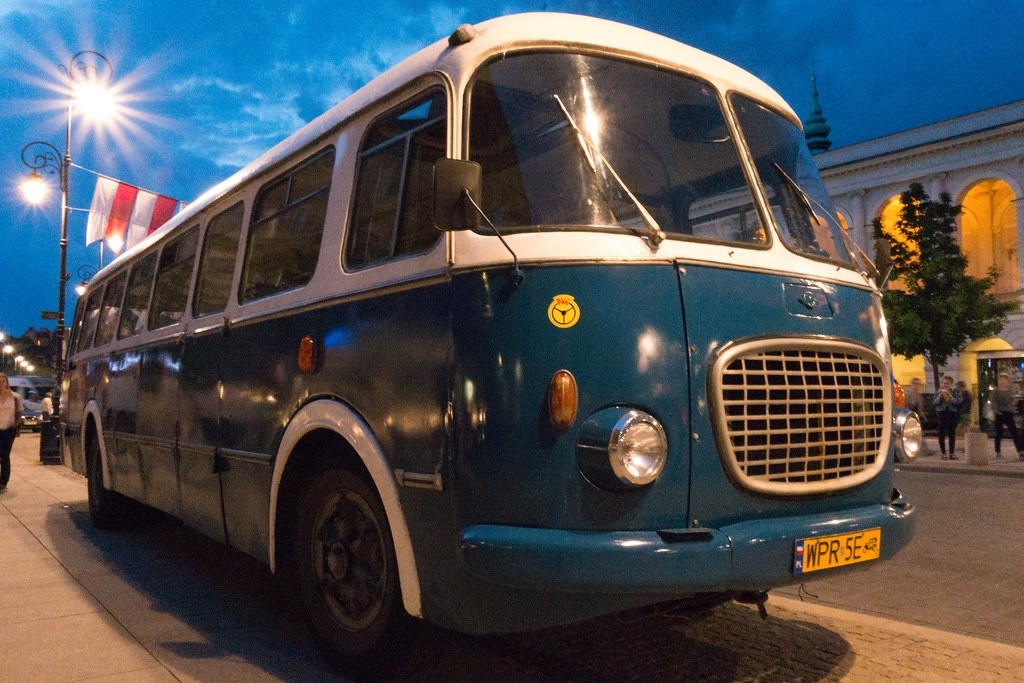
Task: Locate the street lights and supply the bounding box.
[13,44,115,475]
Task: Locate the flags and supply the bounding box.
[87,173,178,255]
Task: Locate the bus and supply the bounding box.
[55,8,927,675]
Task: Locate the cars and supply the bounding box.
[17,403,44,432]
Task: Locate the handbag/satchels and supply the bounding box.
[14,395,20,437]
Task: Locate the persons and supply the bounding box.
[989,373,1024,461]
[904,378,936,457]
[937,378,974,462]
[41,390,55,419]
[0,373,24,493]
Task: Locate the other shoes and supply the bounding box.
[941,454,947,460]
[949,454,959,460]
[0,484,7,492]
[996,451,1004,458]
[1019,451,1024,462]
